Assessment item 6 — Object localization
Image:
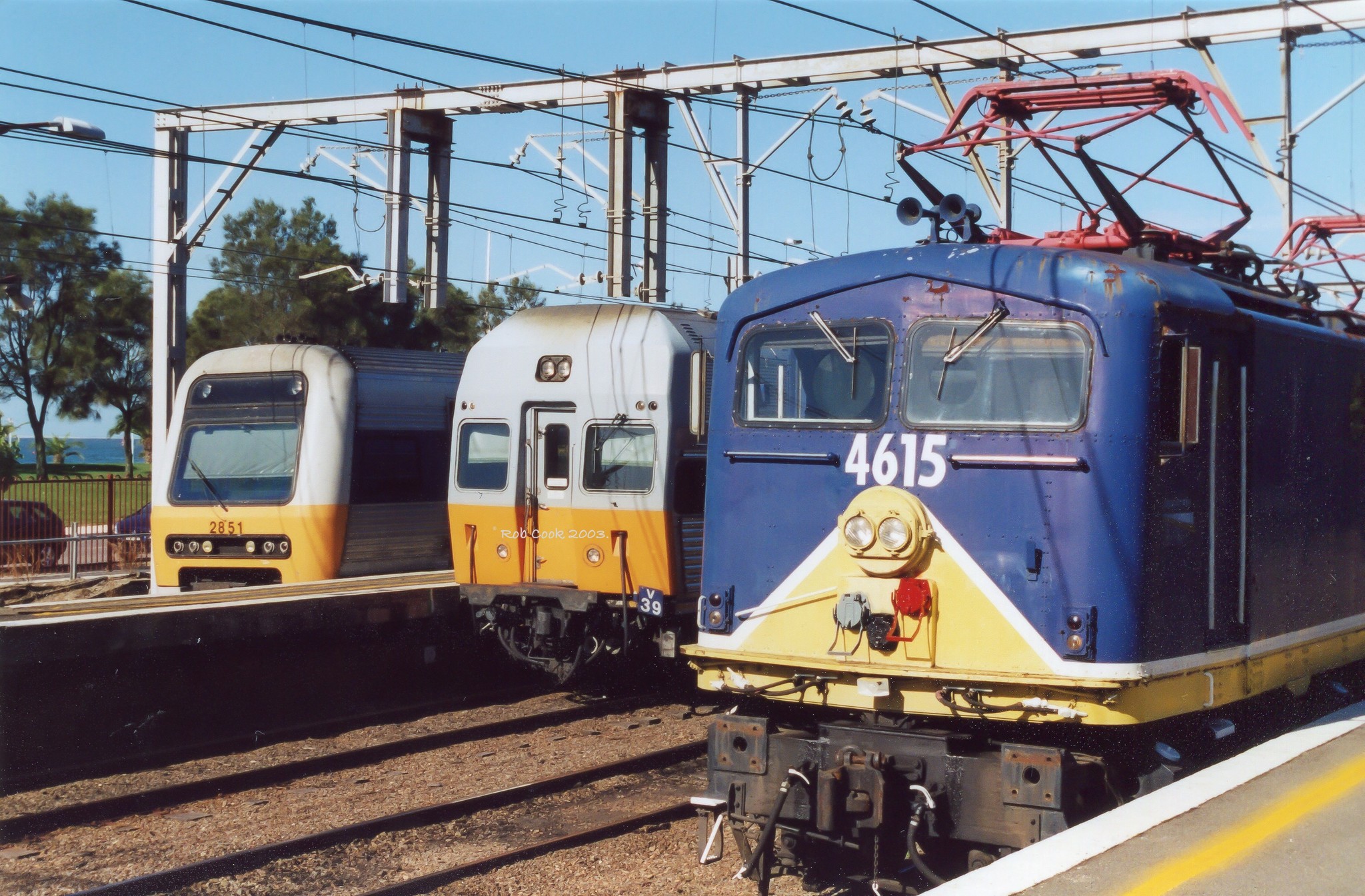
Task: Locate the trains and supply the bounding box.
[442,302,811,702]
[151,335,467,591]
[676,223,1365,896]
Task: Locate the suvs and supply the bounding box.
[0,499,67,573]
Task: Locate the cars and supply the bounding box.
[108,500,150,563]
